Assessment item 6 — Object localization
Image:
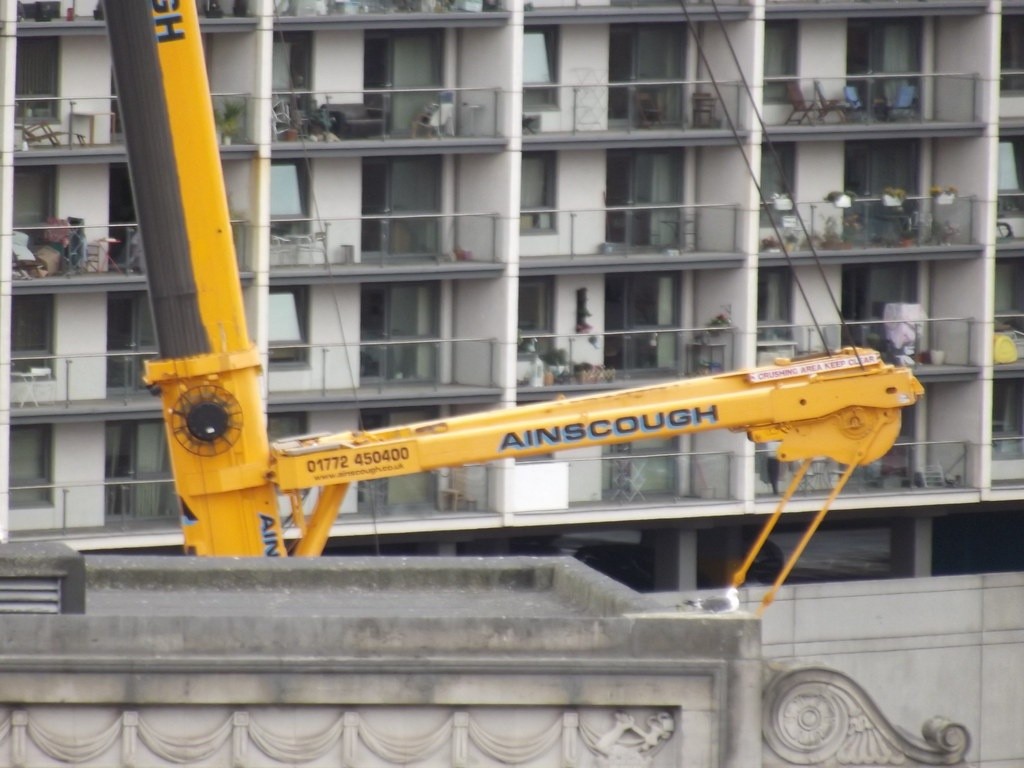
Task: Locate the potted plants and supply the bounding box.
[764,185,957,252]
[210,99,245,146]
[540,347,617,385]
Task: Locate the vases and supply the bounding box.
[932,350,945,365]
[289,129,298,143]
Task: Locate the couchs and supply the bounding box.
[322,101,384,139]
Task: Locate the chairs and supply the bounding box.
[32,368,57,407]
[633,93,664,130]
[782,76,917,125]
[691,93,716,127]
[85,246,100,273]
[793,444,946,493]
[13,121,89,148]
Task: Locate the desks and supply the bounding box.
[757,338,797,369]
[662,219,693,253]
[11,374,42,409]
[68,112,116,147]
[688,341,725,376]
